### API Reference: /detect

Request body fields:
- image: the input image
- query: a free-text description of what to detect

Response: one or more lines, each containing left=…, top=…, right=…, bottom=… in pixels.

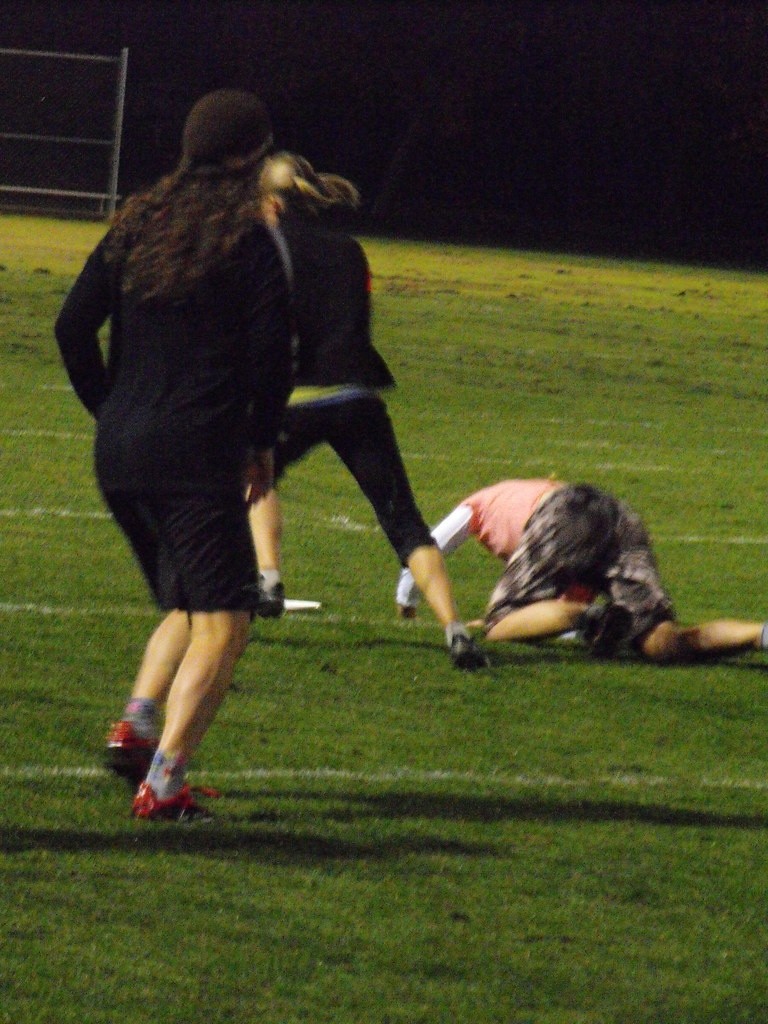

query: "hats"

left=177, top=87, right=275, bottom=180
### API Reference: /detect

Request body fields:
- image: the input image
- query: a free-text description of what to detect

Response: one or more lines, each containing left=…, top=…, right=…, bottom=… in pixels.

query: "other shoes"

left=582, top=605, right=631, bottom=662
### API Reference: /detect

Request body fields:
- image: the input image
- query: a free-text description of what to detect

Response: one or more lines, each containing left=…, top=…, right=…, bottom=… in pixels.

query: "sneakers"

left=105, top=723, right=221, bottom=801
left=129, top=783, right=214, bottom=822
left=257, top=574, right=285, bottom=620
left=450, top=633, right=491, bottom=670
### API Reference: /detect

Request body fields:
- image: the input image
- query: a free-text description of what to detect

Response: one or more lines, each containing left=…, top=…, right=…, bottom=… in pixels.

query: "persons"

left=398, top=477, right=767, bottom=666
left=246, top=150, right=484, bottom=672
left=57, top=89, right=292, bottom=819
